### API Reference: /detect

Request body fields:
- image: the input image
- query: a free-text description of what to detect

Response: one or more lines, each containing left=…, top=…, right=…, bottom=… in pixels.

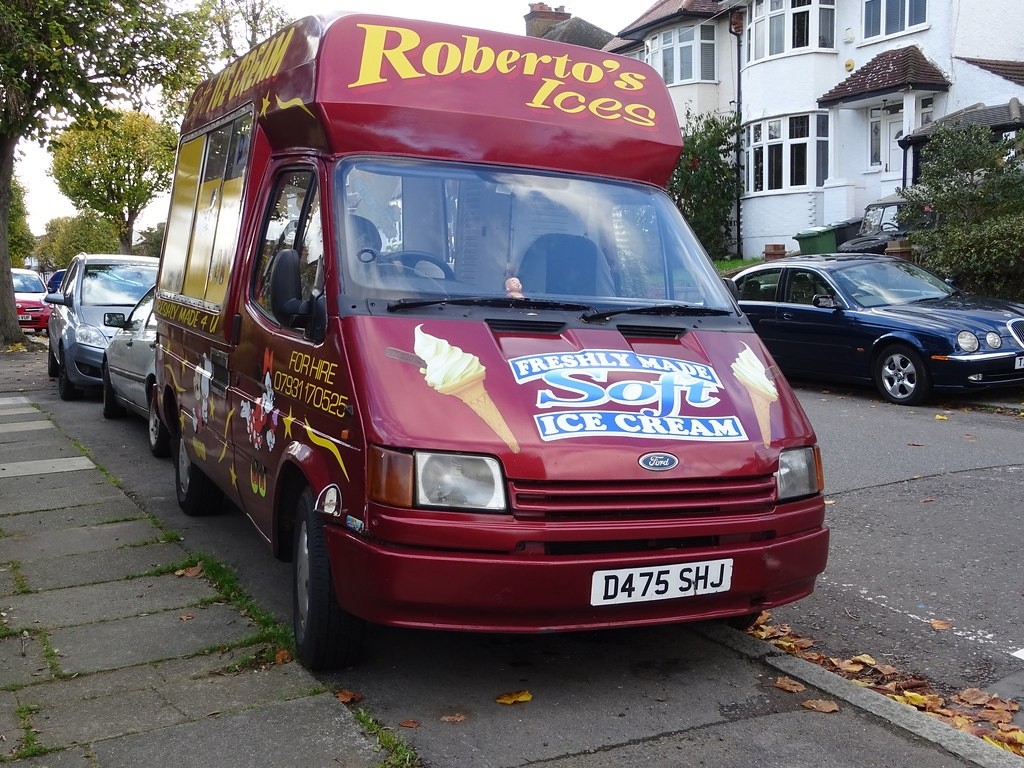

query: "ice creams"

left=385, top=324, right=520, bottom=454
left=730, top=341, right=779, bottom=450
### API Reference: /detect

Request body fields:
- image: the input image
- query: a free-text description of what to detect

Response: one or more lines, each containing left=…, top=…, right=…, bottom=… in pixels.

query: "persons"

left=505, top=278, right=523, bottom=299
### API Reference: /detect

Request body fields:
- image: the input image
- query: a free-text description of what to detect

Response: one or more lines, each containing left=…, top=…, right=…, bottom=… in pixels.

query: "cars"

left=45, top=269, right=67, bottom=294
left=102, top=282, right=177, bottom=457
left=729, top=252, right=1024, bottom=406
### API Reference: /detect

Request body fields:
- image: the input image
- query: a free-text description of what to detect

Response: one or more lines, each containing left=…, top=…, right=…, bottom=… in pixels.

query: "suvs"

left=48, top=251, right=161, bottom=402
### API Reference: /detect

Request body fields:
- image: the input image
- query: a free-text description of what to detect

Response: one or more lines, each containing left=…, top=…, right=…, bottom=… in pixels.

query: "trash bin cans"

left=792, top=224, right=840, bottom=254
left=828, top=216, right=870, bottom=245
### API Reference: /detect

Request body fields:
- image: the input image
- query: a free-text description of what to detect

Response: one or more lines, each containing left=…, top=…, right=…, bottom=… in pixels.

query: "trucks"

left=151, top=12, right=829, bottom=684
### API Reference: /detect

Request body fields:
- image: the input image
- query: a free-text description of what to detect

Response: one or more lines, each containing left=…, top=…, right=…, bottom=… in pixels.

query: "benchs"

left=741, top=280, right=778, bottom=301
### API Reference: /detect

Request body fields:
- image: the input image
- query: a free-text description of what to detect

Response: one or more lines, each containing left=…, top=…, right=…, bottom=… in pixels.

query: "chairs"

left=792, top=274, right=828, bottom=304
left=349, top=215, right=385, bottom=256
left=517, top=235, right=616, bottom=293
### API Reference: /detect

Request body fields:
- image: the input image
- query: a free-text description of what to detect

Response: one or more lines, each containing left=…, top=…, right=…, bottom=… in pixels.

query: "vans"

left=10, top=268, right=51, bottom=332
